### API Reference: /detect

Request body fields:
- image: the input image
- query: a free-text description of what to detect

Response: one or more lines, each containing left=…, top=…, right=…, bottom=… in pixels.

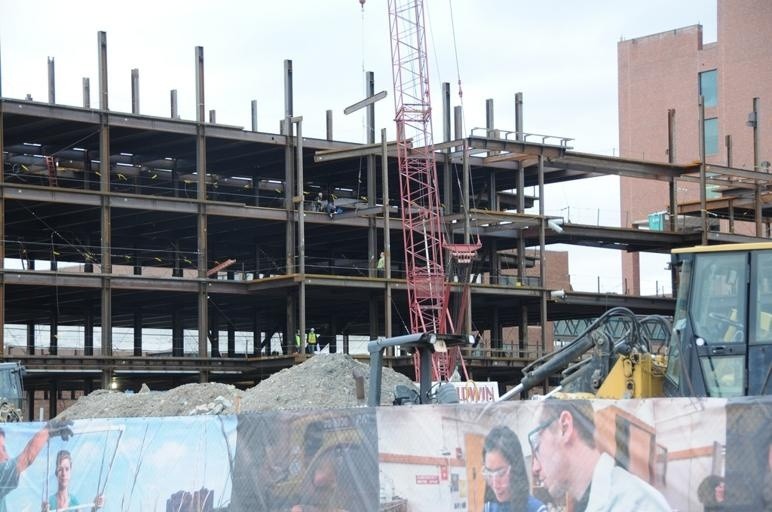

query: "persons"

left=313, top=192, right=323, bottom=212
left=481, top=426, right=547, bottom=512
left=307, top=327, right=318, bottom=353
left=0, top=420, right=73, bottom=512
left=295, top=329, right=300, bottom=353
left=377, top=251, right=385, bottom=278
left=528, top=400, right=670, bottom=512
left=41, top=450, right=104, bottom=512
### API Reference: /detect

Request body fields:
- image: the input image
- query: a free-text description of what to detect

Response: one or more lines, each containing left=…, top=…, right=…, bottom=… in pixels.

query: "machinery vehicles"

left=271, top=329, right=475, bottom=510
left=479, top=242, right=771, bottom=397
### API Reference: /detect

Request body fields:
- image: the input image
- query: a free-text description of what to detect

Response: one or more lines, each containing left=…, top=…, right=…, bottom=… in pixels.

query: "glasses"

left=528, top=416, right=560, bottom=455
left=478, top=467, right=505, bottom=482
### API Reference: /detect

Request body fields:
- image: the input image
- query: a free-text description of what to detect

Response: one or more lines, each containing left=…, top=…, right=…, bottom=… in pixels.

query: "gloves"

left=45, top=421, right=76, bottom=441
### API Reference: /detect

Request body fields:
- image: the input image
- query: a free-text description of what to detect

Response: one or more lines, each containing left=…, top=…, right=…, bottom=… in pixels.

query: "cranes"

left=360, top=0, right=499, bottom=402
